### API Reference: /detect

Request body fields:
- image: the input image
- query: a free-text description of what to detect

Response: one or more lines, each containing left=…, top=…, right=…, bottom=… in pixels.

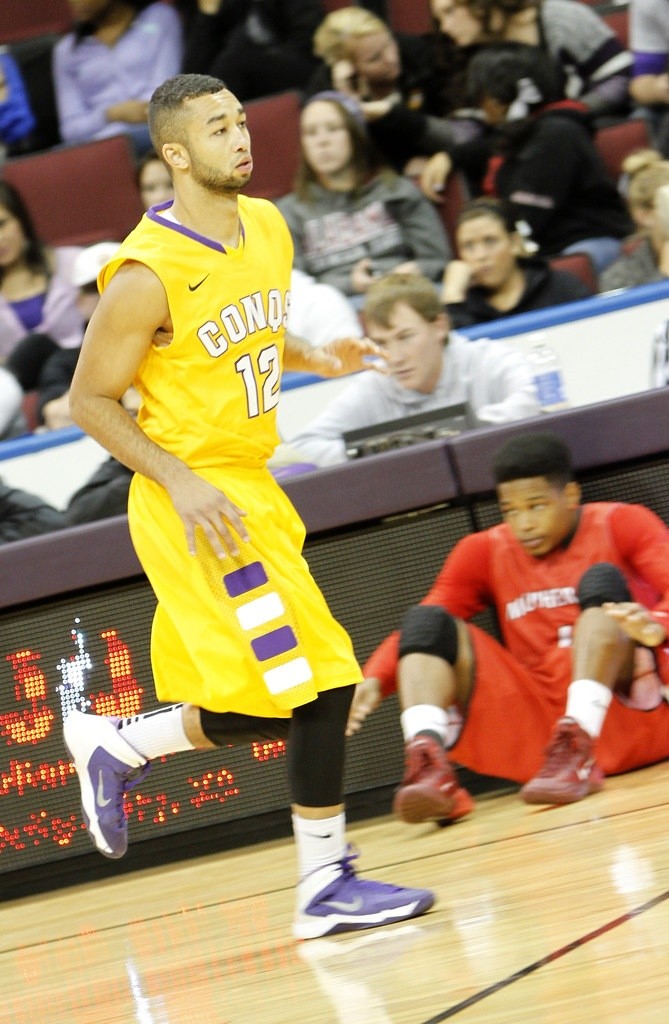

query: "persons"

left=1, top=1, right=669, bottom=541
left=67, top=73, right=435, bottom=943
left=344, top=433, right=668, bottom=826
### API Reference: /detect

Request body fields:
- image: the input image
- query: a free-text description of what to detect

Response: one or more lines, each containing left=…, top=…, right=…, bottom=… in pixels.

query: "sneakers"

left=522, top=716, right=603, bottom=803
left=62, top=711, right=151, bottom=859
left=397, top=737, right=475, bottom=828
left=293, top=843, right=435, bottom=940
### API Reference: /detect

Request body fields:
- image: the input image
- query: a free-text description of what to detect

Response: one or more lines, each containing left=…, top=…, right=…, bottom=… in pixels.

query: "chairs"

left=1, top=0, right=652, bottom=296
